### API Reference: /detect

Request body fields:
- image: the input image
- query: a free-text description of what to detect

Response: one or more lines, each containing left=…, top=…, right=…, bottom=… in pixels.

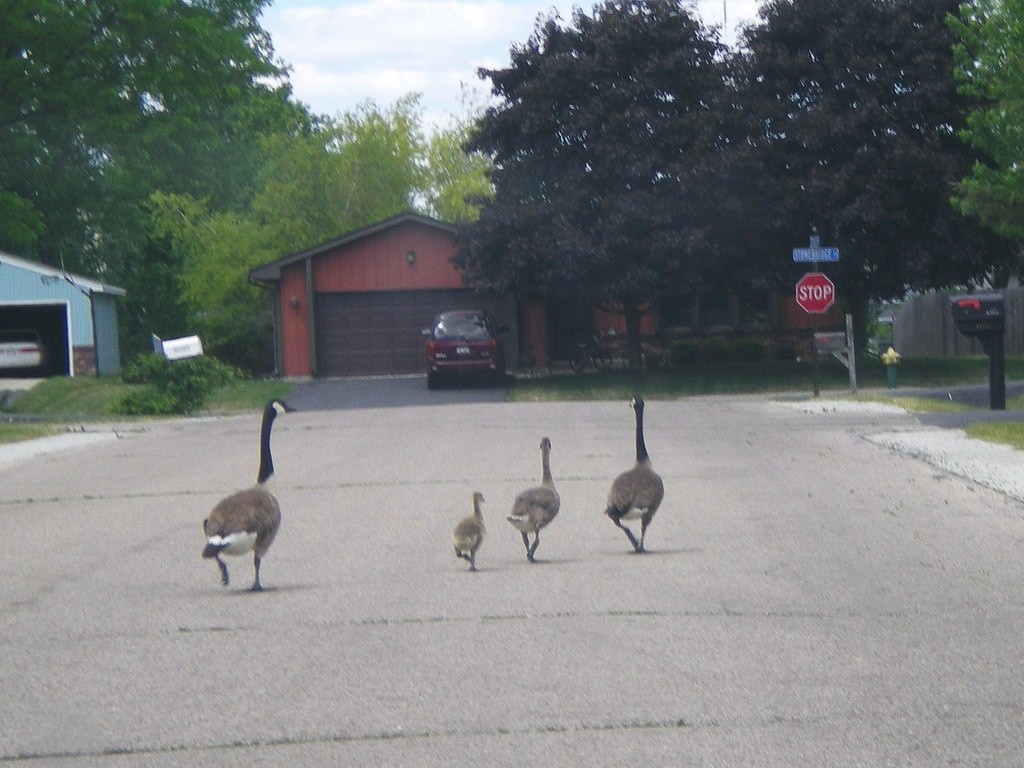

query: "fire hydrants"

left=881, top=346, right=903, bottom=390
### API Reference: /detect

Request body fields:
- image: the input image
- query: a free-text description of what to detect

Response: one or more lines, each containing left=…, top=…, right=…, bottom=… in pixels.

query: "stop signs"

left=795, top=273, right=835, bottom=314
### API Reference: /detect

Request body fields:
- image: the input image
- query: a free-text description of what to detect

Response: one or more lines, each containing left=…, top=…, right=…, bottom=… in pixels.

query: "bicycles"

left=567, top=340, right=612, bottom=374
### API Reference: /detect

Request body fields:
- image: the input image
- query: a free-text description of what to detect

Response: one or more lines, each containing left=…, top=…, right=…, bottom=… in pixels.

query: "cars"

left=0, top=330, right=54, bottom=376
left=421, top=309, right=510, bottom=392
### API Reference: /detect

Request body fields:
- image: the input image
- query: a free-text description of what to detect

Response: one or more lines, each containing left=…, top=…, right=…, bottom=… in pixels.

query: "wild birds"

left=201, top=398, right=296, bottom=593
left=454, top=491, right=487, bottom=573
left=604, top=394, right=665, bottom=554
left=507, top=436, right=561, bottom=564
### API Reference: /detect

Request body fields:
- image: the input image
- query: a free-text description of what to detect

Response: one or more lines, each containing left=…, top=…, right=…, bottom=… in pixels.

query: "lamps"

left=407, top=251, right=414, bottom=265
left=290, top=294, right=298, bottom=309
